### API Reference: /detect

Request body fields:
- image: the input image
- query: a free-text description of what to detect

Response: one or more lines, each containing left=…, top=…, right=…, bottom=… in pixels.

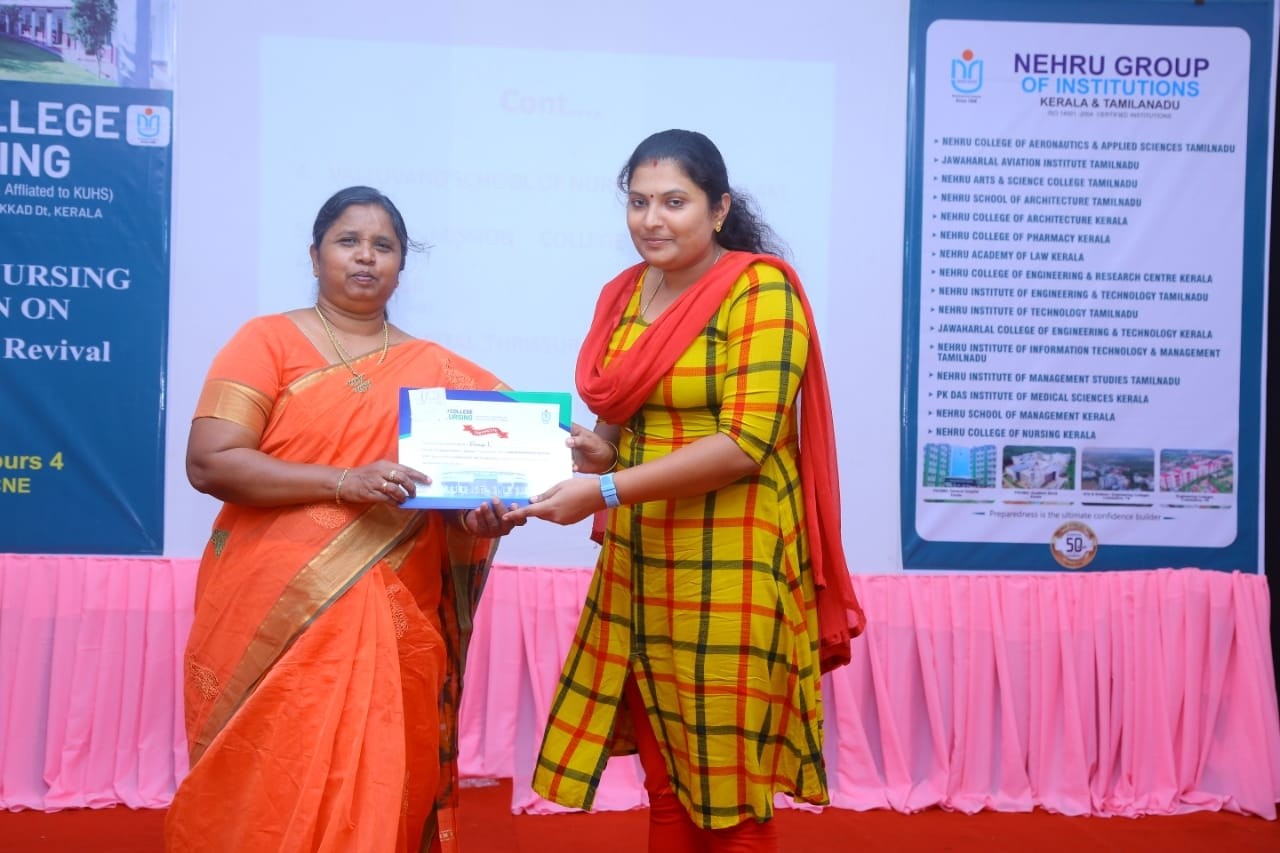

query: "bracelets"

left=335, top=468, right=348, bottom=505
left=600, top=472, right=620, bottom=509
left=459, top=509, right=473, bottom=536
left=600, top=440, right=619, bottom=474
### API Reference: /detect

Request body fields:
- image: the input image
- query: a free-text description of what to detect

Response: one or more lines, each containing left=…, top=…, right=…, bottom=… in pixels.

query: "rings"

left=389, top=470, right=396, bottom=481
left=383, top=481, right=388, bottom=492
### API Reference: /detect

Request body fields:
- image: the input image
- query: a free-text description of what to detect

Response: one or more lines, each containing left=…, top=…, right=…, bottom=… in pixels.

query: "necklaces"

left=314, top=303, right=389, bottom=392
left=329, top=327, right=354, bottom=359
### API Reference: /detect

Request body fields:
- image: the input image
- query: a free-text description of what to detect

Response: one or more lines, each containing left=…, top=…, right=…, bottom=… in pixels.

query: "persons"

left=185, top=186, right=526, bottom=853
left=503, top=131, right=865, bottom=852
